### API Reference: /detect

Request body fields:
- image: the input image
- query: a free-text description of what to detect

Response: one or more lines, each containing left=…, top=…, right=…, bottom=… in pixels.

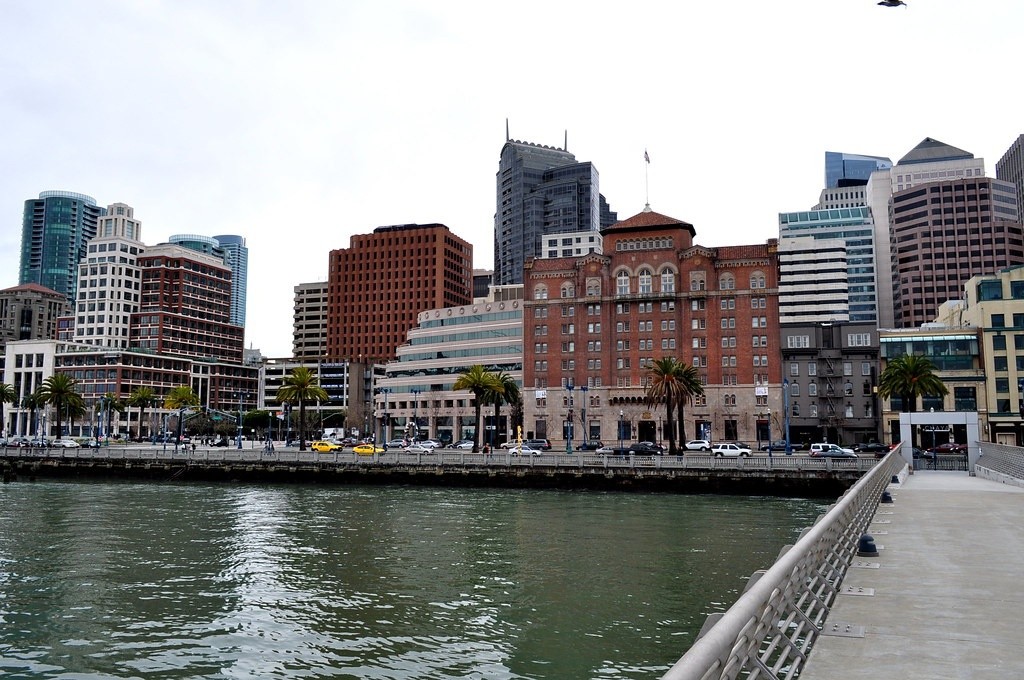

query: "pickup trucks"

left=761, top=440, right=805, bottom=452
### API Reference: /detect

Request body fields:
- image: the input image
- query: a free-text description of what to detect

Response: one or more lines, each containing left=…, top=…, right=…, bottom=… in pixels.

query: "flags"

left=644, top=149, right=651, bottom=164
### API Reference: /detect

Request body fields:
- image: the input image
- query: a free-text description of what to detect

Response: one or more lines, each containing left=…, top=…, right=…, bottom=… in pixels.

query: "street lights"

left=42, top=413, right=46, bottom=445
left=930, top=407, right=937, bottom=470
left=489, top=411, right=493, bottom=458
left=321, top=402, right=343, bottom=434
left=620, top=409, right=624, bottom=461
left=101, top=393, right=118, bottom=447
left=766, top=408, right=773, bottom=468
left=96, top=412, right=101, bottom=450
left=233, top=391, right=251, bottom=449
left=148, top=399, right=164, bottom=446
left=269, top=412, right=272, bottom=450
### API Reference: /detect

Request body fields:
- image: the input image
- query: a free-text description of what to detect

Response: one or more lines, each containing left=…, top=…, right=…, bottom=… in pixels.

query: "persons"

left=17, top=442, right=53, bottom=453
left=180, top=435, right=214, bottom=455
left=483, top=443, right=490, bottom=463
left=98, top=434, right=122, bottom=446
left=676, top=446, right=684, bottom=465
left=263, top=442, right=275, bottom=455
left=598, top=442, right=604, bottom=448
left=383, top=440, right=388, bottom=451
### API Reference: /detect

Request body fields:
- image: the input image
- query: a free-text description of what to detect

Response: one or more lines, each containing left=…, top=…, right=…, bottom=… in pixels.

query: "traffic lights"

left=581, top=386, right=588, bottom=443
left=566, top=385, right=574, bottom=454
left=381, top=417, right=386, bottom=426
left=410, top=389, right=421, bottom=439
left=379, top=387, right=392, bottom=445
left=215, top=412, right=218, bottom=421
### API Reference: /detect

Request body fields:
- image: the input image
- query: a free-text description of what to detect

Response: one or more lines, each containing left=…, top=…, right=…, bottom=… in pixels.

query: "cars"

left=0, top=437, right=100, bottom=448
left=290, top=439, right=552, bottom=457
left=809, top=441, right=967, bottom=464
left=210, top=438, right=228, bottom=447
left=130, top=436, right=190, bottom=444
left=576, top=441, right=753, bottom=460
